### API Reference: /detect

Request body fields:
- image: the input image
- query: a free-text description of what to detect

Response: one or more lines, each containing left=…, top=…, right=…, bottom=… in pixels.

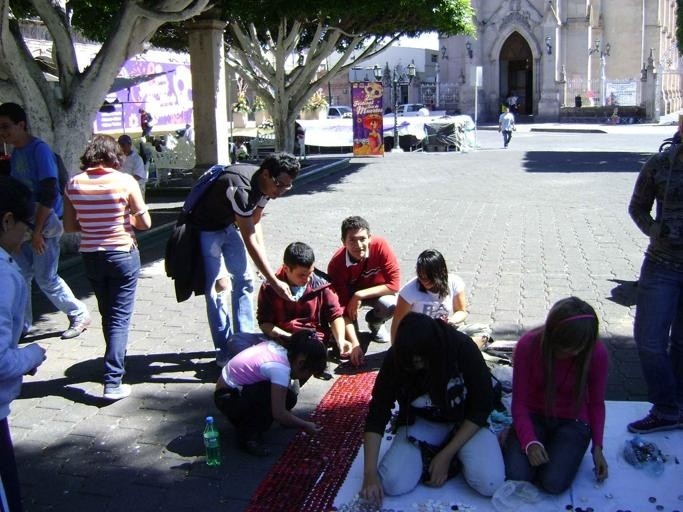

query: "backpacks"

left=54, top=152, right=70, bottom=195
left=179, top=165, right=226, bottom=217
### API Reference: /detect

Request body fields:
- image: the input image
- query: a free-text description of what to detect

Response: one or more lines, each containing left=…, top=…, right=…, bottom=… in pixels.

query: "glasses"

left=272, top=176, right=292, bottom=191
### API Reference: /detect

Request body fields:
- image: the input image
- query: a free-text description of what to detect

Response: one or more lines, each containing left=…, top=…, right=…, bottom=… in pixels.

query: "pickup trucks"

left=382, top=103, right=448, bottom=118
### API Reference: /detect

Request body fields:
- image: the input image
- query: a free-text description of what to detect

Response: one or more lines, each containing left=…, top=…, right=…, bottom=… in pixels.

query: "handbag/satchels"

left=493, top=381, right=512, bottom=412
left=408, top=426, right=463, bottom=484
left=147, top=115, right=157, bottom=127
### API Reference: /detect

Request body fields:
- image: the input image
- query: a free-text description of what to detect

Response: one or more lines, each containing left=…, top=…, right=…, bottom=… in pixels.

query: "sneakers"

left=62, top=315, right=91, bottom=338
left=368, top=322, right=391, bottom=343
left=244, top=441, right=272, bottom=457
left=315, top=368, right=333, bottom=379
left=216, top=348, right=231, bottom=367
left=628, top=412, right=679, bottom=434
left=104, top=383, right=131, bottom=400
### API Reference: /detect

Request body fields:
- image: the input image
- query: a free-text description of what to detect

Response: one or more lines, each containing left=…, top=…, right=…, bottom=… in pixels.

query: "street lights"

left=374, top=62, right=419, bottom=154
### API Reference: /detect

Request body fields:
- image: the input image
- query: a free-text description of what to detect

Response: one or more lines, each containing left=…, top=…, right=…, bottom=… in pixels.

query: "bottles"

left=203, top=416, right=223, bottom=467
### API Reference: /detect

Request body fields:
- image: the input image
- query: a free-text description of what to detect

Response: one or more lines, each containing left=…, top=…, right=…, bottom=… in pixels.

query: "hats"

left=118, top=135, right=132, bottom=144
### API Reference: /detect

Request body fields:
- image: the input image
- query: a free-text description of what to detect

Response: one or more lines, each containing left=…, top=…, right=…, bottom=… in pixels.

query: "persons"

left=182, top=122, right=194, bottom=145
left=63, top=135, right=151, bottom=400
left=0, top=174, right=46, bottom=511
left=325, top=214, right=402, bottom=369
left=507, top=90, right=520, bottom=122
left=212, top=329, right=325, bottom=451
left=626, top=104, right=682, bottom=434
left=137, top=107, right=153, bottom=138
left=389, top=248, right=491, bottom=341
left=233, top=139, right=247, bottom=156
left=255, top=240, right=353, bottom=372
left=0, top=101, right=92, bottom=339
left=164, top=150, right=302, bottom=369
left=498, top=107, right=516, bottom=148
left=357, top=312, right=504, bottom=507
left=499, top=295, right=608, bottom=495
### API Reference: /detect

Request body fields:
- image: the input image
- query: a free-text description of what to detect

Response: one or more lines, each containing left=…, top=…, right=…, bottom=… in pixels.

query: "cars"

left=327, top=105, right=352, bottom=119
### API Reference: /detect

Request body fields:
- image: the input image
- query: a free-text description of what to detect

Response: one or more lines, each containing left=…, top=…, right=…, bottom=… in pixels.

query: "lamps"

left=545, top=37, right=552, bottom=54
left=441, top=45, right=448, bottom=60
left=458, top=71, right=465, bottom=84
left=589, top=39, right=600, bottom=55
left=466, top=40, right=472, bottom=58
left=604, top=42, right=610, bottom=57
